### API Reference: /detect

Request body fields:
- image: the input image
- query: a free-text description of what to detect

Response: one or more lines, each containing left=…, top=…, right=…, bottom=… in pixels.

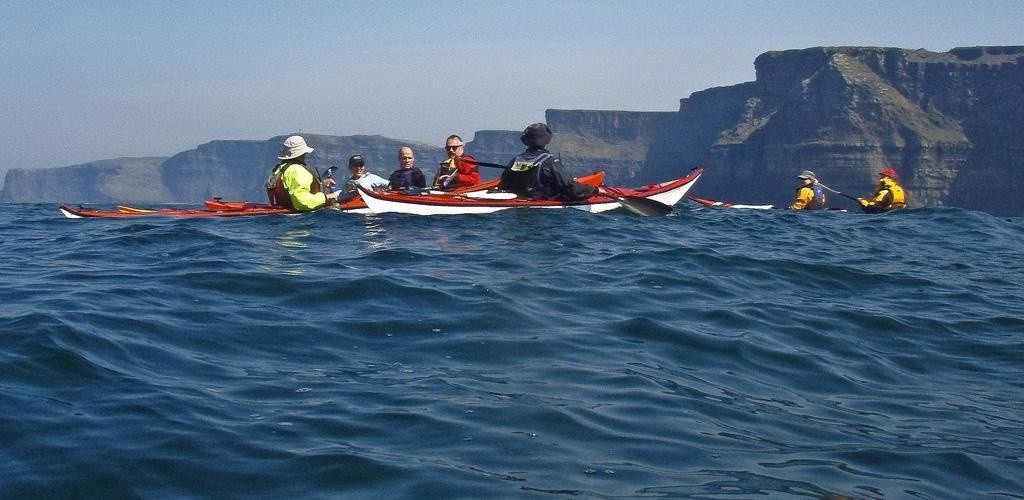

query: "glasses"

left=445, top=143, right=463, bottom=152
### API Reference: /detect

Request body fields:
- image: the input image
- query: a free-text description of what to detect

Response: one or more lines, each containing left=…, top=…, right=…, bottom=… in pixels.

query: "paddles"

left=322, top=165, right=340, bottom=210
left=438, top=169, right=458, bottom=188
left=461, top=159, right=673, bottom=216
left=818, top=183, right=859, bottom=203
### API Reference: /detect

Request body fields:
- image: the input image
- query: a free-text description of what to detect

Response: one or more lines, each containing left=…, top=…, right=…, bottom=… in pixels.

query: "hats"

left=348, top=154, right=364, bottom=164
left=276, top=135, right=314, bottom=160
left=797, top=170, right=816, bottom=180
left=519, top=122, right=553, bottom=148
left=879, top=167, right=898, bottom=181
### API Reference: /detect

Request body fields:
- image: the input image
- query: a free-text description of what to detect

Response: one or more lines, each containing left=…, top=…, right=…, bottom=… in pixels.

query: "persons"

left=336, top=155, right=391, bottom=202
left=265, top=135, right=336, bottom=211
left=431, top=135, right=464, bottom=190
left=786, top=170, right=825, bottom=210
left=387, top=147, right=425, bottom=188
left=857, top=169, right=908, bottom=214
left=499, top=123, right=599, bottom=200
left=438, top=155, right=480, bottom=185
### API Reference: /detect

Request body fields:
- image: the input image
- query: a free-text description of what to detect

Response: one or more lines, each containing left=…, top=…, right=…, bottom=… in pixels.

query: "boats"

left=688, top=193, right=845, bottom=213
